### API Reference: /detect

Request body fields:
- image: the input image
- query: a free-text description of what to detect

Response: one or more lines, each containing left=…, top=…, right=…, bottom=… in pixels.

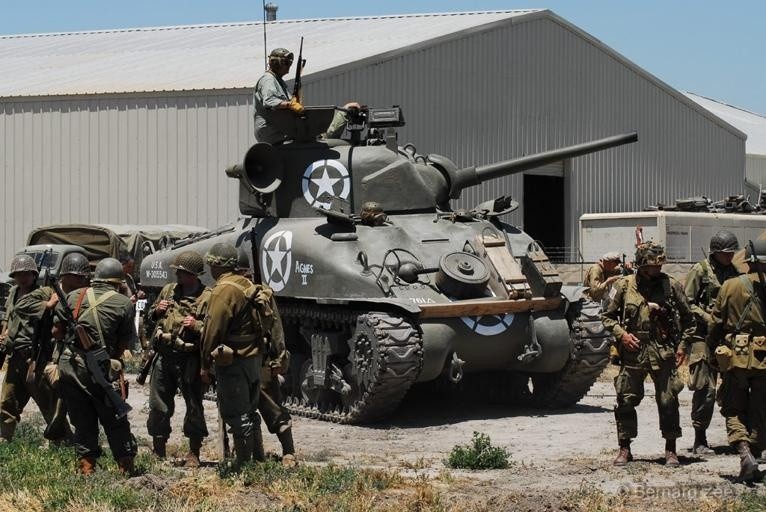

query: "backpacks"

left=217, top=276, right=274, bottom=354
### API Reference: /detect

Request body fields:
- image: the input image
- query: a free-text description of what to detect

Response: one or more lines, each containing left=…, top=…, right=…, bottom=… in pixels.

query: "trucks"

left=0, top=222, right=210, bottom=326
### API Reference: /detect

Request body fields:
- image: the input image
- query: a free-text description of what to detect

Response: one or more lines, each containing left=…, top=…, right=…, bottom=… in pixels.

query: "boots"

left=614, top=437, right=633, bottom=466
left=277, top=426, right=296, bottom=471
left=732, top=440, right=758, bottom=483
left=692, top=429, right=715, bottom=454
left=183, top=437, right=203, bottom=467
left=665, top=439, right=680, bottom=468
left=153, top=434, right=166, bottom=460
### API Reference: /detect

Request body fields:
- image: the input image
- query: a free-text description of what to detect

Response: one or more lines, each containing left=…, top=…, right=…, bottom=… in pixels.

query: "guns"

left=136, top=347, right=156, bottom=384
left=217, top=416, right=231, bottom=461
left=48, top=273, right=133, bottom=420
left=249, top=228, right=281, bottom=395
left=749, top=240, right=766, bottom=299
left=293, top=36, right=306, bottom=101
left=1, top=252, right=47, bottom=368
left=618, top=254, right=626, bottom=326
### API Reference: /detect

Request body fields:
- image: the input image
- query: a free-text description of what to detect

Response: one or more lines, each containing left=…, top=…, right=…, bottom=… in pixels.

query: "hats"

left=602, top=252, right=621, bottom=264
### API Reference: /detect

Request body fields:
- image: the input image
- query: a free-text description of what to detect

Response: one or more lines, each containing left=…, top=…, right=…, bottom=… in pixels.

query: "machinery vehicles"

left=576, top=188, right=766, bottom=263
left=127, top=0, right=640, bottom=428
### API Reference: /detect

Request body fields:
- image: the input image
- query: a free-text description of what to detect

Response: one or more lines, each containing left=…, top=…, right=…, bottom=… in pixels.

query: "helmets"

left=169, top=250, right=205, bottom=276
left=741, top=242, right=752, bottom=263
left=92, top=258, right=123, bottom=284
left=751, top=233, right=766, bottom=264
left=8, top=254, right=39, bottom=281
left=268, top=48, right=293, bottom=69
left=59, top=252, right=90, bottom=276
left=634, top=241, right=666, bottom=267
left=708, top=229, right=739, bottom=255
left=234, top=247, right=250, bottom=270
left=205, top=242, right=237, bottom=268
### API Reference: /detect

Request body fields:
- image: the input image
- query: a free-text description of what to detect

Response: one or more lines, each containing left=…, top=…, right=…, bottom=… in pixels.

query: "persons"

left=0, top=242, right=295, bottom=478
left=583, top=230, right=766, bottom=479
left=321, top=102, right=361, bottom=140
left=253, top=48, right=304, bottom=146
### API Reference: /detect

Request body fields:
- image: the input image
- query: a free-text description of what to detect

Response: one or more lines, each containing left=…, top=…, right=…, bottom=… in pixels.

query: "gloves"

left=289, top=97, right=304, bottom=114
